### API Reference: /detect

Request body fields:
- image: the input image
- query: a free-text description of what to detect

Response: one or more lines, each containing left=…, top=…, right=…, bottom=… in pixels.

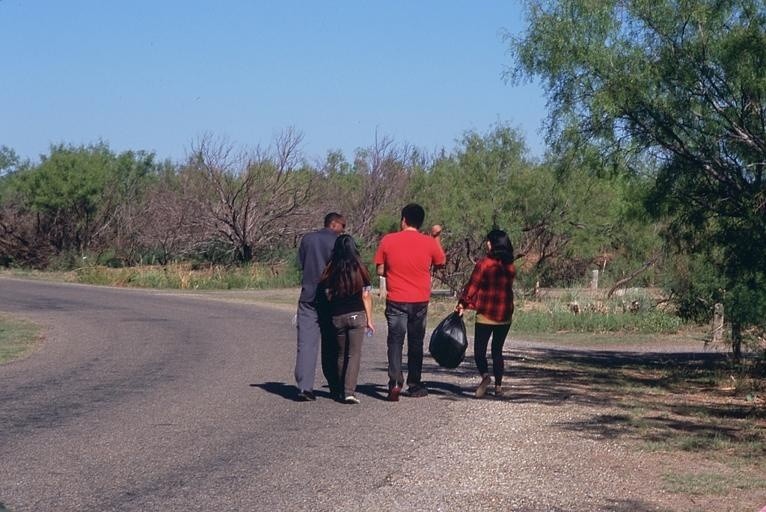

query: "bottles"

left=366, top=327, right=373, bottom=339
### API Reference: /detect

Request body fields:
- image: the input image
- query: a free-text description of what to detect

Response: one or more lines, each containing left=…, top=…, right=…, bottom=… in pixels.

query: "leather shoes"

left=297, top=390, right=316, bottom=401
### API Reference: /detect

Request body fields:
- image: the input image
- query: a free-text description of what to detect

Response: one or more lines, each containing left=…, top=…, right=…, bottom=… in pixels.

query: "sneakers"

left=345, top=395, right=360, bottom=404
left=388, top=379, right=402, bottom=401
left=476, top=376, right=491, bottom=398
left=495, top=390, right=504, bottom=397
left=409, top=387, right=428, bottom=397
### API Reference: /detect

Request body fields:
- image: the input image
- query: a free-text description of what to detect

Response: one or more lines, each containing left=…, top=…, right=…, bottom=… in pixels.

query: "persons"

left=455, top=230, right=515, bottom=398
left=294, top=212, right=345, bottom=401
left=319, top=235, right=375, bottom=403
left=374, top=203, right=447, bottom=401
